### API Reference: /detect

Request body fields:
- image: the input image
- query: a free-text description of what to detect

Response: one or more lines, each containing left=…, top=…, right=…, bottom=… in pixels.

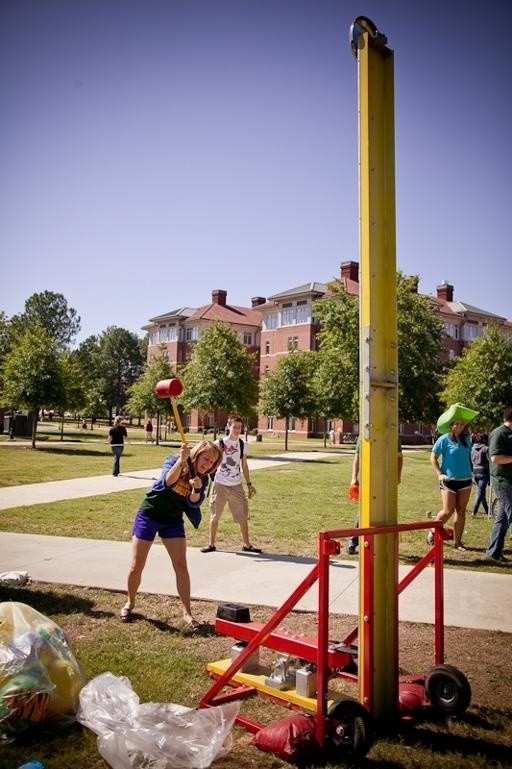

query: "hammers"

left=155, top=379, right=195, bottom=483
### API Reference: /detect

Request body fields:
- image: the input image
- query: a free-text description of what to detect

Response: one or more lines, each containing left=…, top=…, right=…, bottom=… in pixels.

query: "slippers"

left=189, top=620, right=200, bottom=632
left=120, top=608, right=131, bottom=622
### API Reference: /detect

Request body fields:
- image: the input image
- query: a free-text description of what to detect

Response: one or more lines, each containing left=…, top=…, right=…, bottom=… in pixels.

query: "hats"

left=436, top=402, right=478, bottom=434
left=492, top=554, right=507, bottom=562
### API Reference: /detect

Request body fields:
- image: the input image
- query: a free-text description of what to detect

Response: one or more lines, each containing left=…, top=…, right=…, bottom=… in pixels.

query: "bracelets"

left=247, top=483, right=251, bottom=486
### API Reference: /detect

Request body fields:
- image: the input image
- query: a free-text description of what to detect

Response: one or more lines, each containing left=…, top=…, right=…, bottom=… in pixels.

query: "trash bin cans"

left=328, top=430, right=340, bottom=445
left=4, top=415, right=14, bottom=433
left=257, top=434, right=262, bottom=441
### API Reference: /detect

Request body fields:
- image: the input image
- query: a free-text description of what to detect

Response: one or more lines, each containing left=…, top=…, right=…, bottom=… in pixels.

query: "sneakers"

left=454, top=545, right=466, bottom=551
left=242, top=545, right=261, bottom=554
left=347, top=546, right=354, bottom=554
left=427, top=531, right=434, bottom=544
left=201, top=546, right=216, bottom=552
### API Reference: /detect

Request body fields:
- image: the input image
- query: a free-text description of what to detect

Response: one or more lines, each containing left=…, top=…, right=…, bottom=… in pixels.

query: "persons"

left=347, top=415, right=511, bottom=562
left=82, top=419, right=88, bottom=428
left=109, top=417, right=131, bottom=477
left=145, top=420, right=155, bottom=443
left=330, top=429, right=335, bottom=445
left=201, top=416, right=262, bottom=553
left=120, top=440, right=223, bottom=632
